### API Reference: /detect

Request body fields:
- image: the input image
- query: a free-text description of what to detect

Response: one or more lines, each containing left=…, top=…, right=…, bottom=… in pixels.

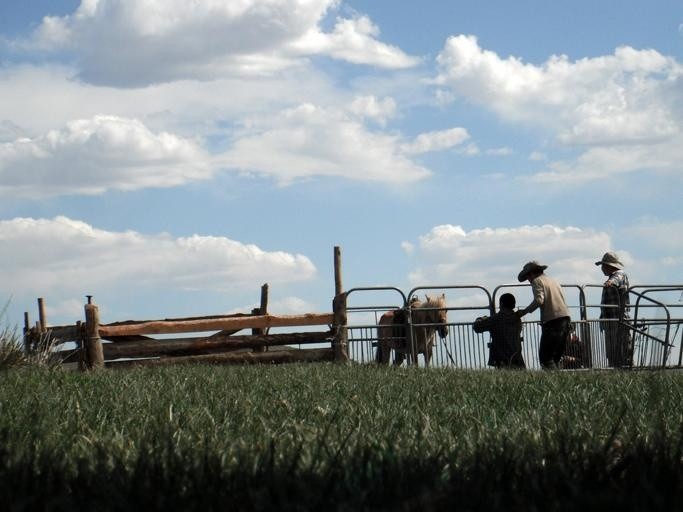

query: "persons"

left=558, top=322, right=585, bottom=369
left=516, top=259, right=571, bottom=369
left=594, top=250, right=635, bottom=368
left=473, top=292, right=527, bottom=368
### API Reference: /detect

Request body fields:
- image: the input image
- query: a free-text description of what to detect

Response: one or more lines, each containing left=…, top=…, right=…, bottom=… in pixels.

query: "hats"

left=594, top=251, right=625, bottom=270
left=517, top=260, right=548, bottom=282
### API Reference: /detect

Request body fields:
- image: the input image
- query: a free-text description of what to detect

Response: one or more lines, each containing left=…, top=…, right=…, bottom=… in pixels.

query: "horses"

left=373, top=292, right=449, bottom=368
